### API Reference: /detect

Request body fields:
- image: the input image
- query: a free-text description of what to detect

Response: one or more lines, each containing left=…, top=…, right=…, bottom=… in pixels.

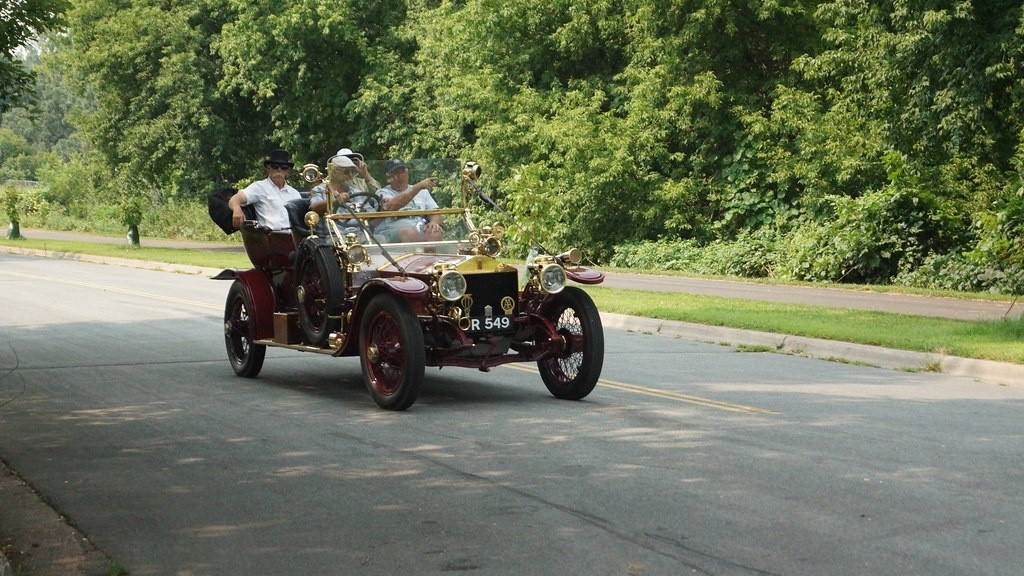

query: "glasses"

left=336, top=168, right=356, bottom=175
left=269, top=163, right=289, bottom=170
left=392, top=168, right=409, bottom=176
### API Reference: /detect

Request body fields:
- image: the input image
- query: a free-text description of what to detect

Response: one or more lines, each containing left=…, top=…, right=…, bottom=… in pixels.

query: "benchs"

left=238, top=190, right=311, bottom=220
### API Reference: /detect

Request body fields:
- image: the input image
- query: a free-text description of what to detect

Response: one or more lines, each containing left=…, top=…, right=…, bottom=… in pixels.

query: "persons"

left=229, top=150, right=303, bottom=308
left=373, top=155, right=446, bottom=243
left=310, top=148, right=389, bottom=244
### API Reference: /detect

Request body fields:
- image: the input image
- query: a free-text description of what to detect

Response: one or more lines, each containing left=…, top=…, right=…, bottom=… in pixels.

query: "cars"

left=208, top=159, right=606, bottom=412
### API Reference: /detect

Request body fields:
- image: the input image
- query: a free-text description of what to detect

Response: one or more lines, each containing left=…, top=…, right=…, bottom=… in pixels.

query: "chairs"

left=283, top=195, right=334, bottom=252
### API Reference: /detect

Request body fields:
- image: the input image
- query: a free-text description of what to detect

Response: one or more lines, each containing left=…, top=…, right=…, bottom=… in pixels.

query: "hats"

left=383, top=158, right=410, bottom=174
left=264, top=149, right=294, bottom=170
left=331, top=156, right=357, bottom=168
left=337, top=149, right=352, bottom=155
